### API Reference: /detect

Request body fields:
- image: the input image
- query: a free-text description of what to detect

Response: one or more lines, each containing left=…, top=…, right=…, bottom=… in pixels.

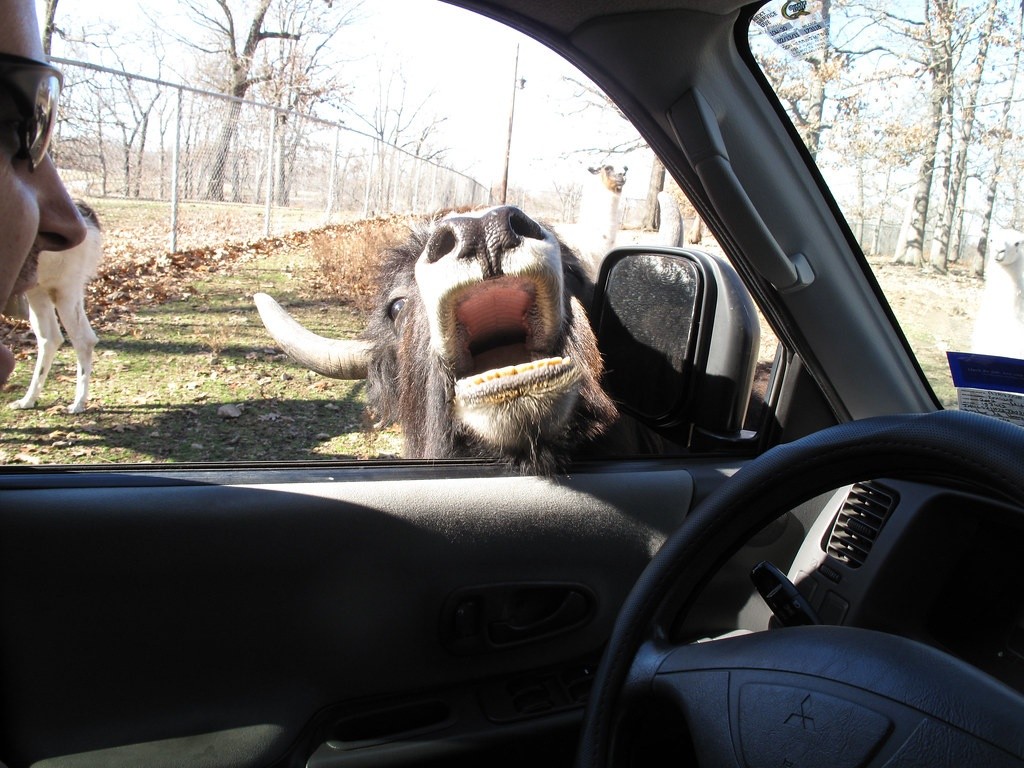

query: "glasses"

left=0, top=52, right=63, bottom=173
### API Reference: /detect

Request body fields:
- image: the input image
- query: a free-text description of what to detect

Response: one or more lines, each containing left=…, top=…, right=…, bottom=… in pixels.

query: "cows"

left=253, top=191, right=683, bottom=478
left=577, top=163, right=629, bottom=251
left=4, top=195, right=106, bottom=414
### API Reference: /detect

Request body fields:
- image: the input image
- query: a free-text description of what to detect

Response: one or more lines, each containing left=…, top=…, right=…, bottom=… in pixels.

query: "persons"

left=0, top=0, right=88, bottom=385
